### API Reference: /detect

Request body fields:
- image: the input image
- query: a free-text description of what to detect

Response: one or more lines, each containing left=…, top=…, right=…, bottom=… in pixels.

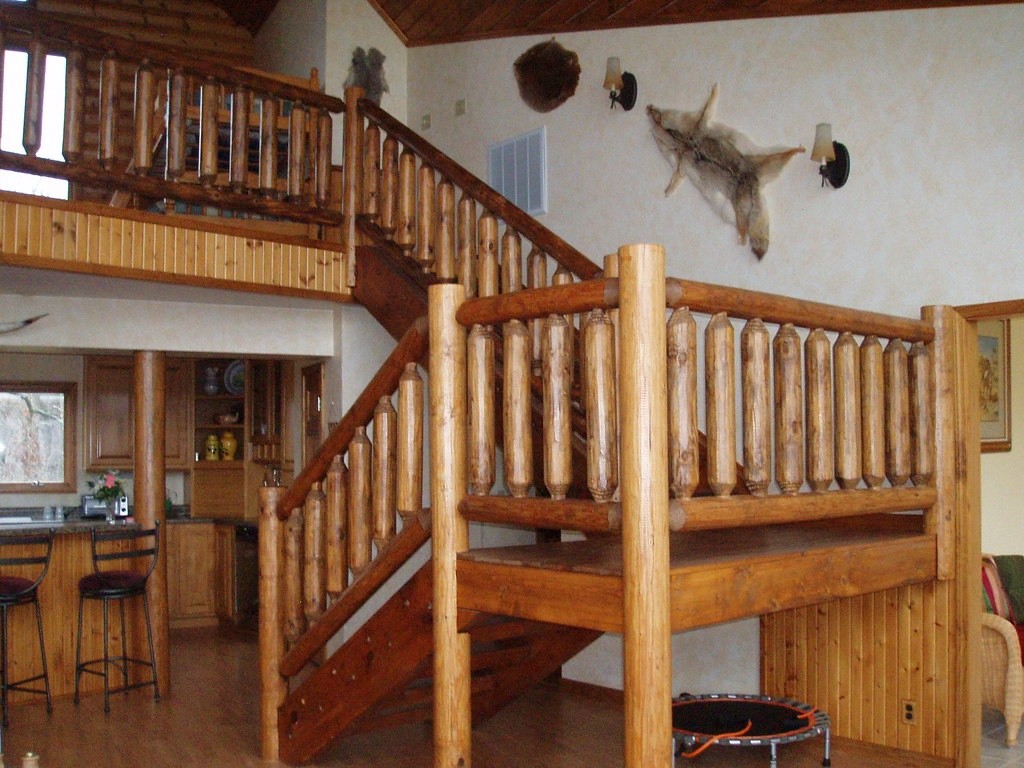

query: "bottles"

left=203, top=436, right=220, bottom=460
left=218, top=430, right=238, bottom=461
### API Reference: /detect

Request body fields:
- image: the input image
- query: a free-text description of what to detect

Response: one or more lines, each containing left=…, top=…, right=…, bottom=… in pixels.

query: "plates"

left=222, top=359, right=248, bottom=395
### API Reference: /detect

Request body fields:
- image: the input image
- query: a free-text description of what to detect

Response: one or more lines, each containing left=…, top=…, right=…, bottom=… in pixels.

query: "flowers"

left=88, top=472, right=128, bottom=506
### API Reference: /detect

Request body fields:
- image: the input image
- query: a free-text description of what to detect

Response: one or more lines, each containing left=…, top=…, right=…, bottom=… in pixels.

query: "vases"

left=105, top=505, right=116, bottom=525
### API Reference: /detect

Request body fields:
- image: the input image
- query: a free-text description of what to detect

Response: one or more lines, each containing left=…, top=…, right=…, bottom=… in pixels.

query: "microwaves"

left=82, top=494, right=128, bottom=516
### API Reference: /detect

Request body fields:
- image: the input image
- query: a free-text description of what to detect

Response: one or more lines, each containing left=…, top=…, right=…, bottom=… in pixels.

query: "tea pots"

left=213, top=413, right=240, bottom=425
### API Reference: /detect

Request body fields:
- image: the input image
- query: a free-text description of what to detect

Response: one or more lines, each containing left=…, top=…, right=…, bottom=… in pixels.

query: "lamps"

left=603, top=56, right=637, bottom=111
left=809, top=123, right=851, bottom=188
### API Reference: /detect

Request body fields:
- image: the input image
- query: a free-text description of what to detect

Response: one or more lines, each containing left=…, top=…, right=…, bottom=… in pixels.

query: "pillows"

left=994, top=555, right=1024, bottom=625
left=982, top=556, right=1016, bottom=625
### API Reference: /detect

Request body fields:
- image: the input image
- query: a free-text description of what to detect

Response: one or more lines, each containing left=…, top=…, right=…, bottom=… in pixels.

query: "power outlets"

left=902, top=700, right=918, bottom=725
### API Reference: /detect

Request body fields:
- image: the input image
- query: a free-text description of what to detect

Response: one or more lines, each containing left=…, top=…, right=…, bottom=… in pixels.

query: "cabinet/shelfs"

left=81, top=353, right=294, bottom=630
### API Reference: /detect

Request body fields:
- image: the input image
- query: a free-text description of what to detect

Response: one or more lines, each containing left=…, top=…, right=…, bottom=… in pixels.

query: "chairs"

left=72, top=522, right=163, bottom=714
left=981, top=553, right=1024, bottom=747
left=0, top=527, right=56, bottom=730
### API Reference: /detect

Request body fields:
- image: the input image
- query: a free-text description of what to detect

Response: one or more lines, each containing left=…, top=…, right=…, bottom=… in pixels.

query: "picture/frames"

left=973, top=318, right=1013, bottom=453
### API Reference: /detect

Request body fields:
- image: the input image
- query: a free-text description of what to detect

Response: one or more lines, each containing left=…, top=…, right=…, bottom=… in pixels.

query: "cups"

left=53, top=505, right=65, bottom=522
left=43, top=506, right=55, bottom=521
